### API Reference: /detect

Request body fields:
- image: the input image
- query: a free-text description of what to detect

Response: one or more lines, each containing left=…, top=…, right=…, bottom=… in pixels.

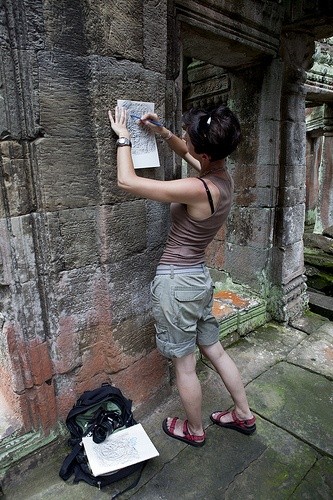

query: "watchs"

left=115, top=137, right=133, bottom=150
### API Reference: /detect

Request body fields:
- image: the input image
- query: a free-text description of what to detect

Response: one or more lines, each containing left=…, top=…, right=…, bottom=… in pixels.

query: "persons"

left=108, top=103, right=256, bottom=449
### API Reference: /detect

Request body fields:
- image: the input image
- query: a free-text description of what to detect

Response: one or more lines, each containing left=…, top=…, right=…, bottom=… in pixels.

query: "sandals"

left=210, top=410, right=256, bottom=434
left=162, top=417, right=206, bottom=446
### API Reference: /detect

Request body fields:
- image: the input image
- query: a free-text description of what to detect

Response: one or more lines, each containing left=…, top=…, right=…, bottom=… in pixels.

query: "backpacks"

left=59, top=383, right=147, bottom=500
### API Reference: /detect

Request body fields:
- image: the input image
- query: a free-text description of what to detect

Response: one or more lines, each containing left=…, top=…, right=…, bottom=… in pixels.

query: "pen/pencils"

left=130, top=115, right=163, bottom=127
